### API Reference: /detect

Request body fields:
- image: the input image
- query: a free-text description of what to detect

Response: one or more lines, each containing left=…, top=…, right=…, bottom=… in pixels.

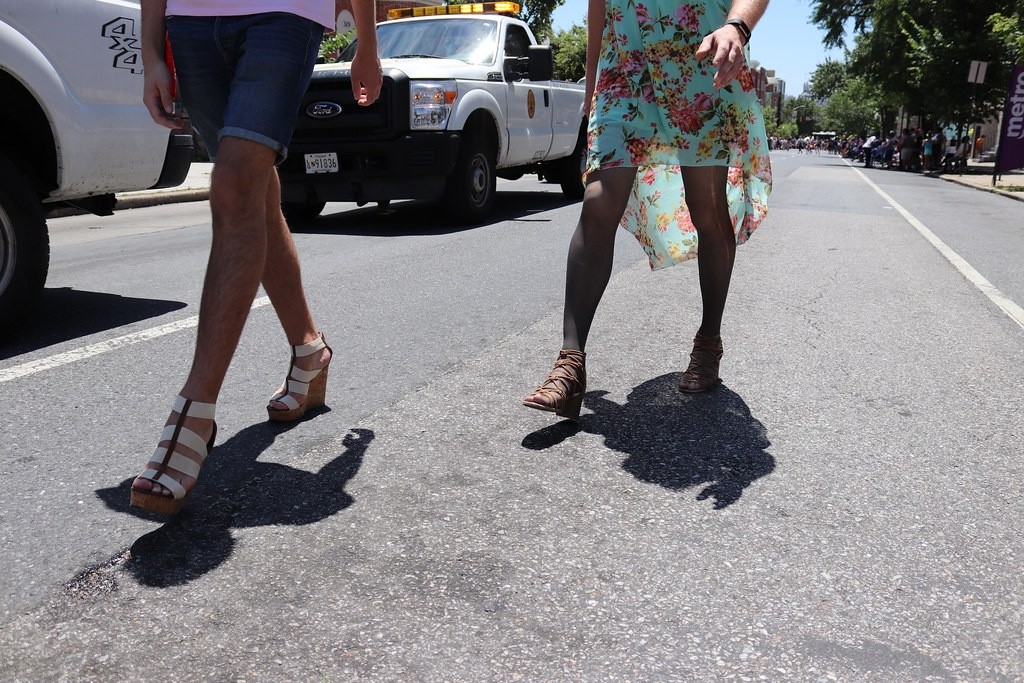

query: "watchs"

left=725, top=19, right=751, bottom=47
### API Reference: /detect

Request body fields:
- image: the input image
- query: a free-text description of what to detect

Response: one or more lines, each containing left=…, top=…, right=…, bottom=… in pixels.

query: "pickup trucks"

left=271, top=1, right=591, bottom=231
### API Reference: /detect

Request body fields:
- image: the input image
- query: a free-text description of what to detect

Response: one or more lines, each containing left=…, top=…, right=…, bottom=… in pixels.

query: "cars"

left=0, top=1, right=194, bottom=348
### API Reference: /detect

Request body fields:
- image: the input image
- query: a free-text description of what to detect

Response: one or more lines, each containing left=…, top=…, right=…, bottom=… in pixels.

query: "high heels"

left=127, top=394, right=217, bottom=518
left=680, top=331, right=724, bottom=392
left=268, top=336, right=333, bottom=420
left=524, top=349, right=588, bottom=417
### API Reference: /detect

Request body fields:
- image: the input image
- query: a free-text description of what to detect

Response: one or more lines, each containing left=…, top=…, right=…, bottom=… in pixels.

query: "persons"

left=764, top=126, right=986, bottom=174
left=128, top=0, right=387, bottom=516
left=523, top=0, right=771, bottom=416
